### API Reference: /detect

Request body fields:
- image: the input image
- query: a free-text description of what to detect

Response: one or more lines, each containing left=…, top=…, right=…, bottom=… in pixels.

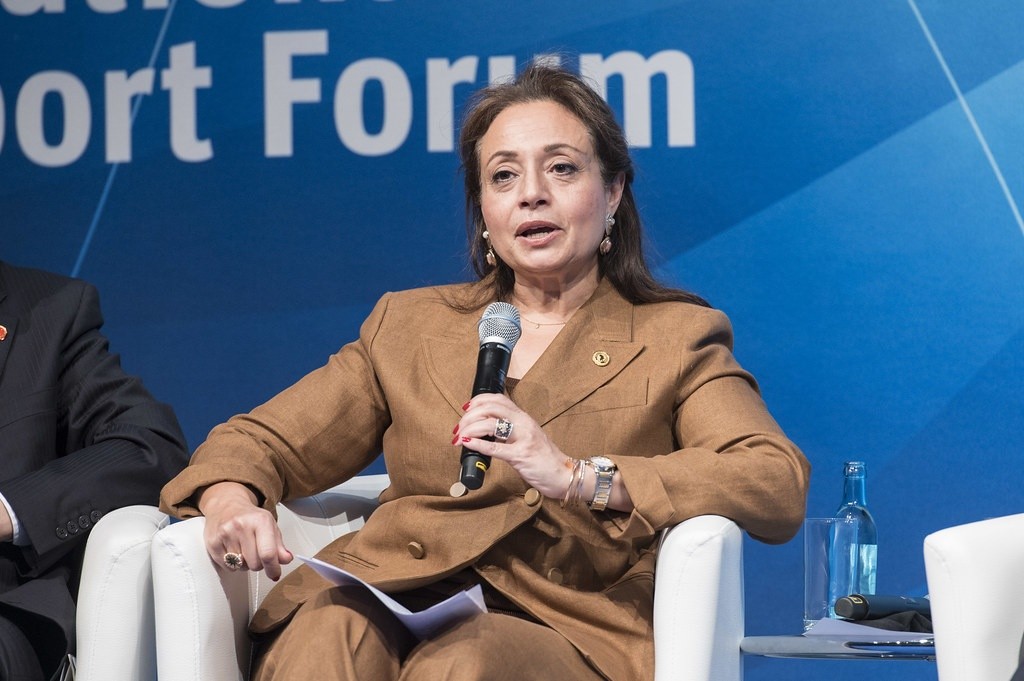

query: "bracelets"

left=560, top=459, right=587, bottom=508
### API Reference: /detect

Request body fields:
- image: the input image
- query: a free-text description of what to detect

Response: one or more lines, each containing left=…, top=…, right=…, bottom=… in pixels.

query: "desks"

left=742, top=635, right=936, bottom=663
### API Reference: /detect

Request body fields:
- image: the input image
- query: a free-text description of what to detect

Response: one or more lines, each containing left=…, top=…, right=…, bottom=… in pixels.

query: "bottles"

left=828, top=461, right=879, bottom=620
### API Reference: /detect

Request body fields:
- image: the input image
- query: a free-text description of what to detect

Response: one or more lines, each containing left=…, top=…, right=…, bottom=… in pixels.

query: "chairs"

left=151, top=474, right=745, bottom=681
left=75, top=506, right=170, bottom=681
left=923, top=512, right=1024, bottom=681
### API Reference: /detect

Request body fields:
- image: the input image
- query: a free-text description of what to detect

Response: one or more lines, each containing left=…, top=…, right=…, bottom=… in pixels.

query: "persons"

left=159, top=67, right=811, bottom=681
left=0, top=261, right=190, bottom=681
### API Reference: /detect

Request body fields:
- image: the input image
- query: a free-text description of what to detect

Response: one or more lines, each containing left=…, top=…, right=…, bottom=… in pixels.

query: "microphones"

left=835, top=593, right=932, bottom=621
left=460, top=302, right=522, bottom=490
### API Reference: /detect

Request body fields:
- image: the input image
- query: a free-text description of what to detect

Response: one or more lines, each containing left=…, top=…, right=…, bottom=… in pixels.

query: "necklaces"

left=521, top=316, right=566, bottom=328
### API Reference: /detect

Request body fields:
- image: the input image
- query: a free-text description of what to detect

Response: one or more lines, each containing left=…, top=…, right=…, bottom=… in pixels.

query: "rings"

left=224, top=553, right=243, bottom=569
left=494, top=418, right=513, bottom=439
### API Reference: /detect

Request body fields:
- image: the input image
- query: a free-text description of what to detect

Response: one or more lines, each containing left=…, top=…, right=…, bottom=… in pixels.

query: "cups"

left=803, top=517, right=858, bottom=632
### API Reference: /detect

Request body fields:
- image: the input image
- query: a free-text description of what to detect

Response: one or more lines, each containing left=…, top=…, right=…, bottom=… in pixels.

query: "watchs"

left=587, top=455, right=617, bottom=511
